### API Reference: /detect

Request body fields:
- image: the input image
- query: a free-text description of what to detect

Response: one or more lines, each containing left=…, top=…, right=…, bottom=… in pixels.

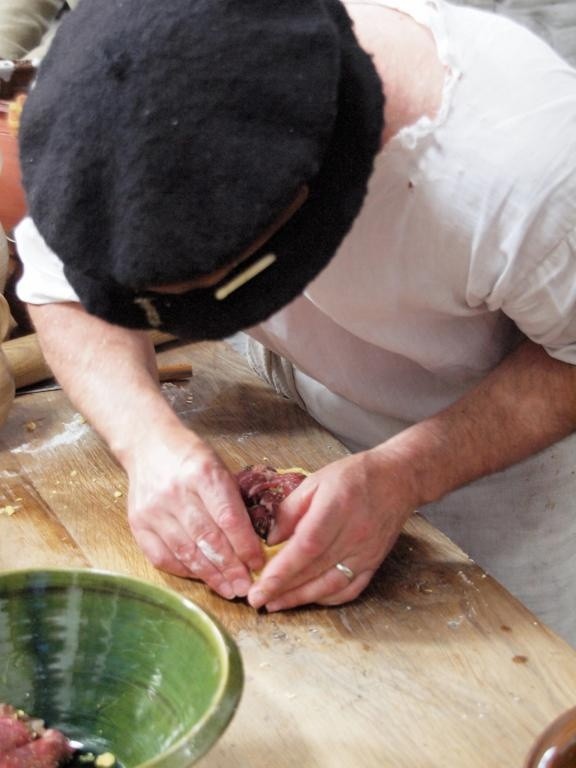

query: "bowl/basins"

left=0, top=567, right=243, bottom=768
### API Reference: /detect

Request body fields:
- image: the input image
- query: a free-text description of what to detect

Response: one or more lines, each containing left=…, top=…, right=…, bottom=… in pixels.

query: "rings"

left=336, top=562, right=355, bottom=581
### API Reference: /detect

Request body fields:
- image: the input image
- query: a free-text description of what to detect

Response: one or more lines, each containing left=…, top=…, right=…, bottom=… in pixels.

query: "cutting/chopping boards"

left=0, top=336, right=576, bottom=768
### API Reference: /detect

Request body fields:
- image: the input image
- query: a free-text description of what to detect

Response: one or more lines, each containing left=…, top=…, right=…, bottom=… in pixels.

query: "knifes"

left=14, top=363, right=192, bottom=395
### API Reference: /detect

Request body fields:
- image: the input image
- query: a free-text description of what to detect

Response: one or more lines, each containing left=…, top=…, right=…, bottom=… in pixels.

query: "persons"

left=13, top=0, right=576, bottom=610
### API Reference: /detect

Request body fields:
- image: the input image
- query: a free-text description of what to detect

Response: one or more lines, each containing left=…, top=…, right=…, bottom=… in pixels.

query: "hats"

left=14, top=1, right=388, bottom=339
